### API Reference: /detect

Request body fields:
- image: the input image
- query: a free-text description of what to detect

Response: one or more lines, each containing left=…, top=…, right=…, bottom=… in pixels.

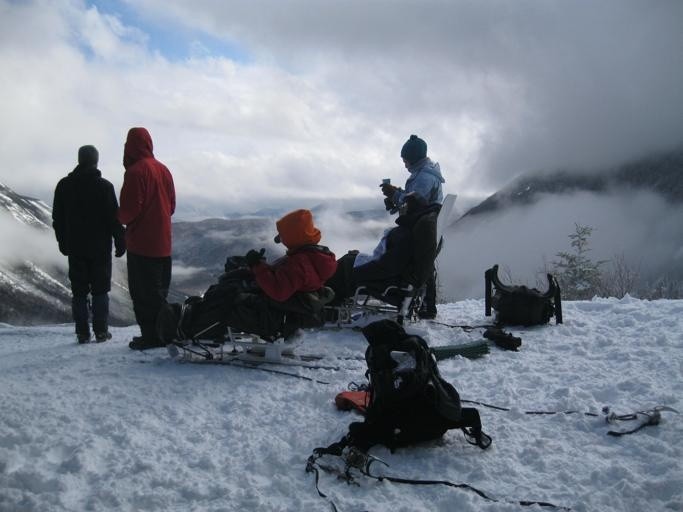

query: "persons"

left=163, top=210, right=338, bottom=345
left=117, top=127, right=176, bottom=350
left=381, top=135, right=445, bottom=319
left=322, top=190, right=431, bottom=322
left=52, top=145, right=127, bottom=344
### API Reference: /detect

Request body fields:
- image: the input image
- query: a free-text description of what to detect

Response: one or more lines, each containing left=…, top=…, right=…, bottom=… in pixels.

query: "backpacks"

left=344, top=319, right=481, bottom=449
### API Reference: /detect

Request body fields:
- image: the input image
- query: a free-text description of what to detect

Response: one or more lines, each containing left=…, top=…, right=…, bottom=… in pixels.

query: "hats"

left=401, top=134, right=428, bottom=164
left=78, top=144, right=101, bottom=164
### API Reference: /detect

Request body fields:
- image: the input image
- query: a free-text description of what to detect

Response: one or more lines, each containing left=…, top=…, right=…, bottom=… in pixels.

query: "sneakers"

left=418, top=304, right=439, bottom=320
left=129, top=332, right=171, bottom=350
left=76, top=330, right=112, bottom=343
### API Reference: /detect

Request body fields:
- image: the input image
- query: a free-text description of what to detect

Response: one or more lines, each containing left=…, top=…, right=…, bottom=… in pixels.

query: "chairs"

left=353, top=235, right=445, bottom=312
left=162, top=288, right=335, bottom=362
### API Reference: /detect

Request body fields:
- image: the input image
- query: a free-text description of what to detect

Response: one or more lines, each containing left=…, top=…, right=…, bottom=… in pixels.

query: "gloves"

left=244, top=249, right=266, bottom=269
left=58, top=241, right=70, bottom=257
left=379, top=183, right=399, bottom=216
left=113, top=239, right=126, bottom=258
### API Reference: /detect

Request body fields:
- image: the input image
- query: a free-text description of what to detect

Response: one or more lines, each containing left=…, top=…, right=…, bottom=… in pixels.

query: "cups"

left=263, top=344, right=281, bottom=362
left=381, top=178, right=389, bottom=185
